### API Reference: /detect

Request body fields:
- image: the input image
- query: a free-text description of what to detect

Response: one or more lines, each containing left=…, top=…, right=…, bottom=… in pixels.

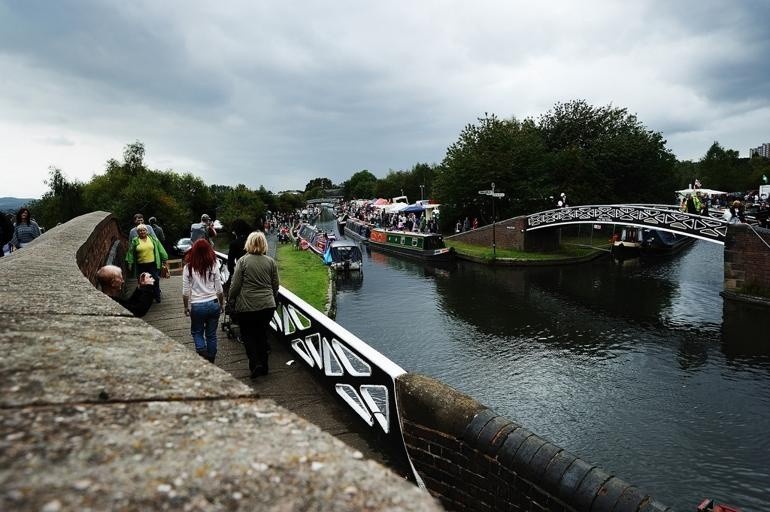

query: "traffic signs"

left=492, top=193, right=505, bottom=197
left=479, top=190, right=493, bottom=195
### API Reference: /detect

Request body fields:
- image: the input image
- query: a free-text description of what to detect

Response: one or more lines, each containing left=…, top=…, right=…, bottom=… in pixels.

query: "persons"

left=1, top=208, right=43, bottom=257
left=224, top=218, right=273, bottom=357
left=455, top=219, right=462, bottom=234
left=125, top=213, right=159, bottom=247
left=95, top=264, right=157, bottom=319
left=147, top=216, right=167, bottom=246
left=472, top=217, right=479, bottom=232
left=262, top=204, right=319, bottom=251
left=224, top=231, right=281, bottom=380
left=180, top=238, right=226, bottom=364
left=678, top=189, right=770, bottom=229
left=122, top=223, right=169, bottom=304
left=560, top=192, right=569, bottom=209
left=334, top=197, right=441, bottom=233
left=190, top=213, right=217, bottom=247
left=463, top=217, right=470, bottom=232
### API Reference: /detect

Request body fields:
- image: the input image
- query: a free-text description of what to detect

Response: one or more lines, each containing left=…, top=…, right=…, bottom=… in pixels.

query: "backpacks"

left=190, top=224, right=206, bottom=244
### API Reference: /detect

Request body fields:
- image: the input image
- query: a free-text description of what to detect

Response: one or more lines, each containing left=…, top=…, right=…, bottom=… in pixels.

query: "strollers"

left=220, top=275, right=243, bottom=340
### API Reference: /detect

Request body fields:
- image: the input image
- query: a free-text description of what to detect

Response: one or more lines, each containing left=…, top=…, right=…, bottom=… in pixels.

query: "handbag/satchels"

left=158, top=265, right=171, bottom=279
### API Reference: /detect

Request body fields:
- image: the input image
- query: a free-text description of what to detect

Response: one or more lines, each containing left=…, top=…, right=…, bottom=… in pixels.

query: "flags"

left=695, top=179, right=702, bottom=188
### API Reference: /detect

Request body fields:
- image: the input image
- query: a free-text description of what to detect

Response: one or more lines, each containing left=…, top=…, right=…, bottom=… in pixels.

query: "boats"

left=334, top=269, right=364, bottom=293
left=295, top=214, right=457, bottom=271
left=612, top=224, right=697, bottom=253
left=613, top=250, right=651, bottom=270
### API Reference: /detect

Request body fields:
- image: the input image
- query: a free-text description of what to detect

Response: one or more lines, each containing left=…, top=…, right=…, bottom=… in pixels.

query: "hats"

left=202, top=213, right=211, bottom=219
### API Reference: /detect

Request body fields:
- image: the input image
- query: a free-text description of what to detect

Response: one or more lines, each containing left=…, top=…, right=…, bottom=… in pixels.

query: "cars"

left=173, top=238, right=193, bottom=256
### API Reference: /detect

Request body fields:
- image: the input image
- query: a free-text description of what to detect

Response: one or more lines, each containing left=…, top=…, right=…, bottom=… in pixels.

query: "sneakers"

left=250, top=360, right=269, bottom=379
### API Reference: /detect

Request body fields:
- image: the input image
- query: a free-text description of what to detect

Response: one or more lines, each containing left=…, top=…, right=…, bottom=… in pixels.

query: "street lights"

left=420, top=185, right=424, bottom=200
